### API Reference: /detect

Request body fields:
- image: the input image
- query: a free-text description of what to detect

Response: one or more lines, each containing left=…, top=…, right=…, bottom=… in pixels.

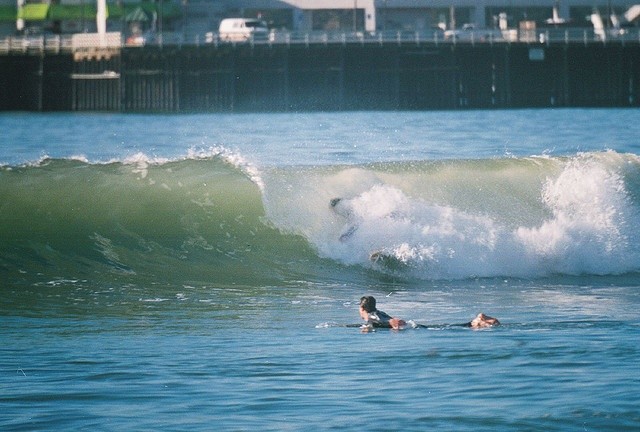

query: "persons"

left=358, top=295, right=500, bottom=331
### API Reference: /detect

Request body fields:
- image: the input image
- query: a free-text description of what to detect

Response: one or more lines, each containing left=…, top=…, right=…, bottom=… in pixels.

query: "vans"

left=217, top=17, right=272, bottom=46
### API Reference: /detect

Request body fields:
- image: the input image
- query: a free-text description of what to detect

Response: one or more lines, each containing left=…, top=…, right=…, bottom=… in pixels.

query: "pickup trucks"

left=443, top=22, right=504, bottom=43
